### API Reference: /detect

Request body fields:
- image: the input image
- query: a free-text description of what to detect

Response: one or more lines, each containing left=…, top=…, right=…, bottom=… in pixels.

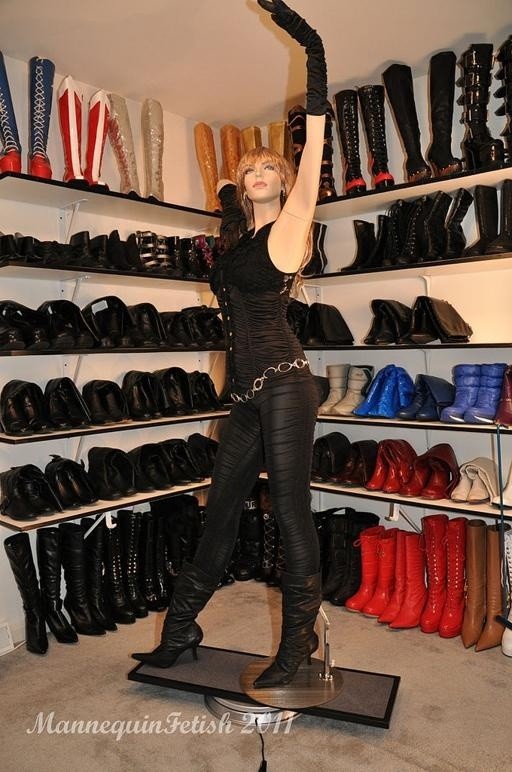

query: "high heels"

left=0, top=231, right=73, bottom=266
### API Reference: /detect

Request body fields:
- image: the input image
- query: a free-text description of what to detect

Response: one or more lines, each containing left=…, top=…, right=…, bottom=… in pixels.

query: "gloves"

left=218, top=183, right=248, bottom=251
left=258, top=0, right=327, bottom=116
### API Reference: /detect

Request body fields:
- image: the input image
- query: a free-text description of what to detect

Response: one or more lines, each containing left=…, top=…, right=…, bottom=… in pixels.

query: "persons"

left=130, top=0, right=329, bottom=690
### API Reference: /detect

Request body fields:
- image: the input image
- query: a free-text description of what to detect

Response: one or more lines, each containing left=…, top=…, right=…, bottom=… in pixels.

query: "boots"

left=194, top=122, right=223, bottom=214
left=252, top=570, right=322, bottom=689
left=342, top=188, right=475, bottom=272
left=428, top=51, right=462, bottom=177
left=335, top=90, right=366, bottom=196
left=301, top=219, right=328, bottom=278
left=483, top=179, right=512, bottom=255
left=455, top=44, right=504, bottom=170
left=287, top=105, right=306, bottom=176
left=285, top=300, right=354, bottom=346
left=106, top=94, right=141, bottom=198
left=28, top=56, right=53, bottom=181
left=0, top=296, right=226, bottom=350
left=0, top=51, right=21, bottom=175
left=493, top=34, right=512, bottom=160
left=57, top=75, right=89, bottom=190
left=140, top=98, right=163, bottom=203
left=220, top=124, right=262, bottom=199
left=0, top=433, right=219, bottom=520
left=314, top=363, right=512, bottom=427
left=131, top=559, right=218, bottom=668
left=463, top=185, right=498, bottom=257
left=311, top=432, right=512, bottom=508
left=311, top=507, right=512, bottom=658
left=70, top=230, right=221, bottom=278
left=0, top=367, right=230, bottom=437
left=318, top=100, right=337, bottom=201
left=410, top=295, right=473, bottom=345
left=268, top=121, right=294, bottom=163
left=364, top=298, right=413, bottom=345
left=382, top=64, right=431, bottom=183
left=84, top=89, right=109, bottom=194
left=358, top=85, right=394, bottom=190
